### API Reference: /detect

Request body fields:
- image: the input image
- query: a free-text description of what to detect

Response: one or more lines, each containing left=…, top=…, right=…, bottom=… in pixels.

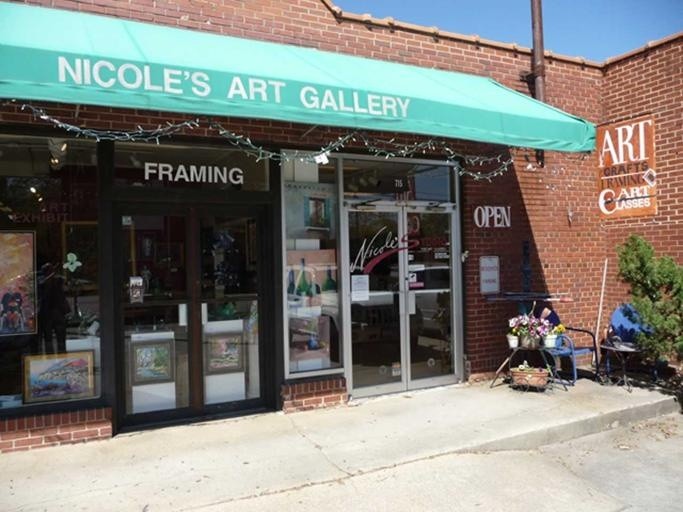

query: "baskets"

left=509, top=367, right=549, bottom=388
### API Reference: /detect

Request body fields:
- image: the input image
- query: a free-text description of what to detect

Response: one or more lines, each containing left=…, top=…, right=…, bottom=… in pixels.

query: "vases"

left=510, top=367, right=548, bottom=391
left=506, top=332, right=561, bottom=349
left=64, top=287, right=84, bottom=324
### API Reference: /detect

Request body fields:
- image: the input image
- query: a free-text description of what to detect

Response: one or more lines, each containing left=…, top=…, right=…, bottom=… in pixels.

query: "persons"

left=31, top=245, right=73, bottom=360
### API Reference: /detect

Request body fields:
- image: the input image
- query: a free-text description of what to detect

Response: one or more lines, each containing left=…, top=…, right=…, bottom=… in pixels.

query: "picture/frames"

left=131, top=331, right=245, bottom=387
left=0, top=228, right=38, bottom=336
left=247, top=219, right=257, bottom=265
left=21, top=349, right=95, bottom=404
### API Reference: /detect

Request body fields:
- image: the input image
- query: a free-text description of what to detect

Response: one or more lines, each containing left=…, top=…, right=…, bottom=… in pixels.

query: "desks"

left=490, top=347, right=569, bottom=393
left=593, top=342, right=647, bottom=394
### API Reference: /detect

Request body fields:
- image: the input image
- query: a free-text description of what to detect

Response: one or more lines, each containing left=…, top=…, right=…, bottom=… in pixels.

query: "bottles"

left=287, top=257, right=337, bottom=308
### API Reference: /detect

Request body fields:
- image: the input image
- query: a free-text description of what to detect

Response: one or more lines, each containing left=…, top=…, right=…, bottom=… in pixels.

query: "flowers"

left=504, top=312, right=567, bottom=335
left=62, top=252, right=85, bottom=313
left=518, top=360, right=545, bottom=372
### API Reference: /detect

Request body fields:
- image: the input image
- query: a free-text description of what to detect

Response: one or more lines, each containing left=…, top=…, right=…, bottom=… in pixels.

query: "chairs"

left=509, top=302, right=599, bottom=394
left=604, top=303, right=658, bottom=382
